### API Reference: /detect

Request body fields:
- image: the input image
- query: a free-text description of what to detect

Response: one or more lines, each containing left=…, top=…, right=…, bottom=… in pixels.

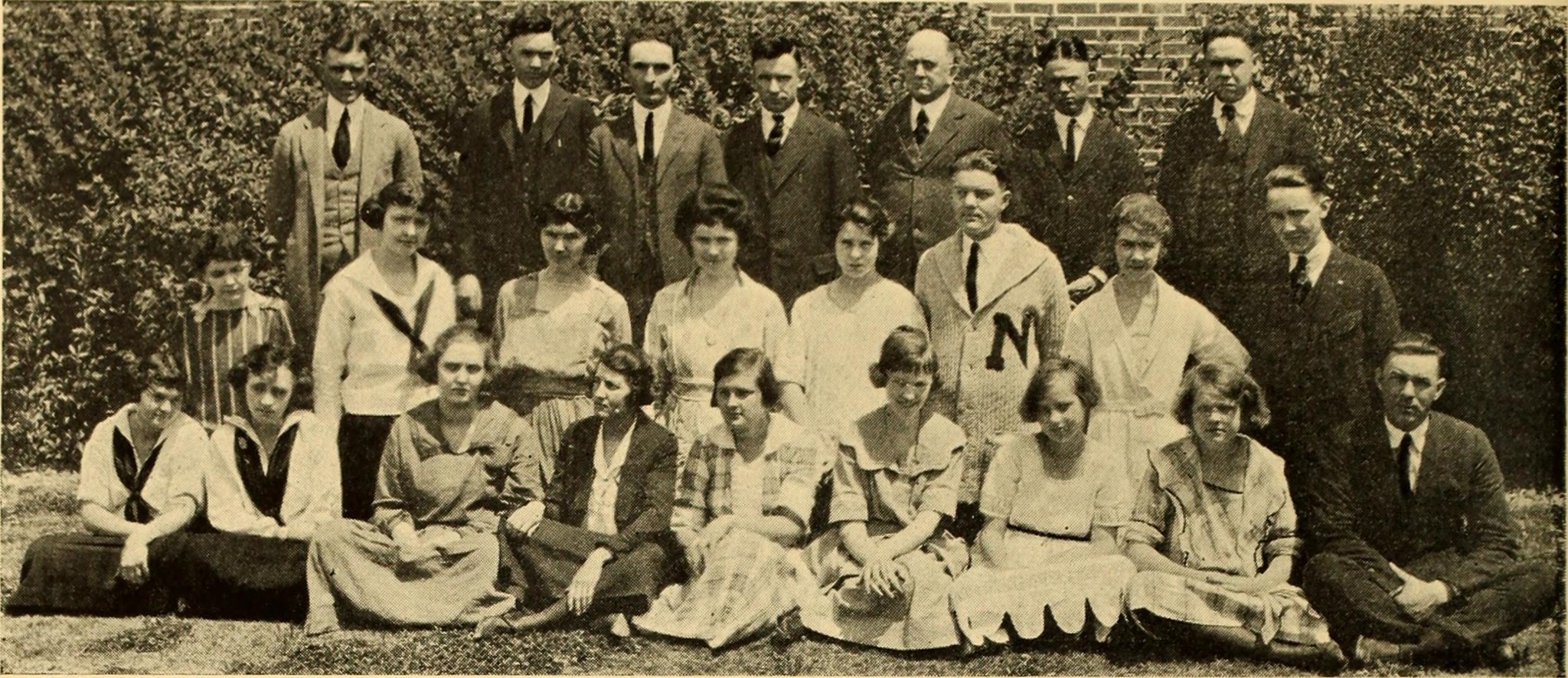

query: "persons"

left=800, top=324, right=970, bottom=651
left=304, top=325, right=542, bottom=638
left=584, top=33, right=726, bottom=347
left=1219, top=165, right=1400, bottom=502
left=474, top=343, right=677, bottom=641
left=452, top=16, right=598, bottom=333
left=725, top=35, right=859, bottom=317
left=311, top=180, right=455, bottom=523
left=1298, top=329, right=1560, bottom=670
left=863, top=28, right=1008, bottom=293
left=788, top=197, right=931, bottom=446
left=157, top=343, right=341, bottom=620
left=267, top=29, right=423, bottom=348
left=1121, top=357, right=1346, bottom=672
left=1060, top=192, right=1252, bottom=500
left=1156, top=26, right=1318, bottom=325
left=915, top=149, right=1072, bottom=542
left=165, top=228, right=299, bottom=434
left=642, top=181, right=789, bottom=496
left=5, top=352, right=208, bottom=615
left=951, top=358, right=1138, bottom=644
left=634, top=349, right=820, bottom=651
left=1004, top=35, right=1148, bottom=309
left=488, top=191, right=635, bottom=487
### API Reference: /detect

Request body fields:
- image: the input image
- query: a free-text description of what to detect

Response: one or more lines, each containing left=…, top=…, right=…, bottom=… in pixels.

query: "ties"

left=964, top=243, right=980, bottom=313
left=1395, top=433, right=1414, bottom=495
left=331, top=107, right=351, bottom=172
left=521, top=94, right=533, bottom=136
left=766, top=114, right=785, bottom=159
left=1222, top=105, right=1245, bottom=160
left=642, top=112, right=653, bottom=167
left=912, top=108, right=931, bottom=146
left=1066, top=119, right=1078, bottom=170
left=1288, top=255, right=1312, bottom=306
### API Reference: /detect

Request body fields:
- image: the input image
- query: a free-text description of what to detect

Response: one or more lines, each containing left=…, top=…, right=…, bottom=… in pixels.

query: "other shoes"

left=1452, top=642, right=1516, bottom=670
left=961, top=630, right=997, bottom=658
left=1348, top=633, right=1398, bottom=668
left=590, top=611, right=631, bottom=639
left=472, top=613, right=519, bottom=640
left=1308, top=636, right=1346, bottom=675
left=775, top=611, right=809, bottom=643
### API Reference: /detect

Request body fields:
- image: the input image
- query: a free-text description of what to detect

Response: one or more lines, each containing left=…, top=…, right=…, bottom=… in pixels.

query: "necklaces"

left=694, top=270, right=738, bottom=346
